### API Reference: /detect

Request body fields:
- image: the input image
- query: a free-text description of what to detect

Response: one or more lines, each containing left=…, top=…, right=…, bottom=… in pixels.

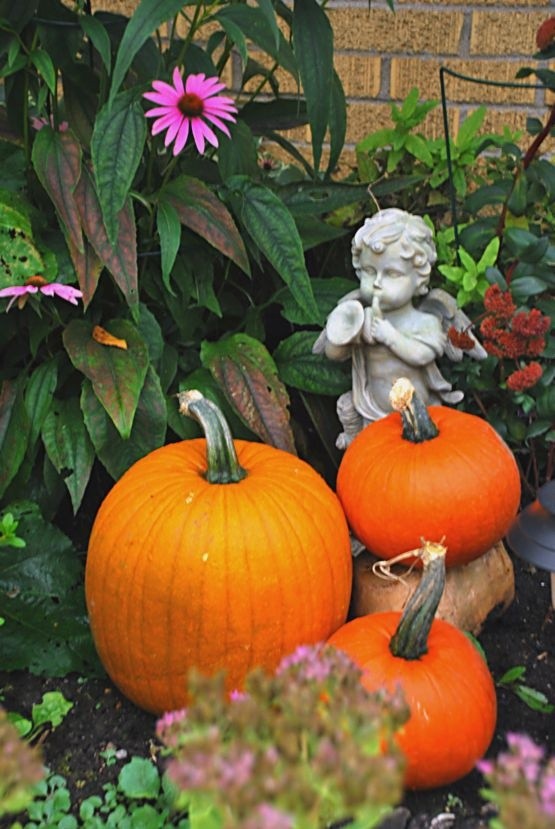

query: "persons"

left=312, top=208, right=487, bottom=448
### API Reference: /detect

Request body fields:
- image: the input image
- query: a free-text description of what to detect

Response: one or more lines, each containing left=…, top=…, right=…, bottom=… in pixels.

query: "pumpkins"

left=336, top=378, right=521, bottom=569
left=82, top=388, right=352, bottom=715
left=308, top=540, right=497, bottom=790
